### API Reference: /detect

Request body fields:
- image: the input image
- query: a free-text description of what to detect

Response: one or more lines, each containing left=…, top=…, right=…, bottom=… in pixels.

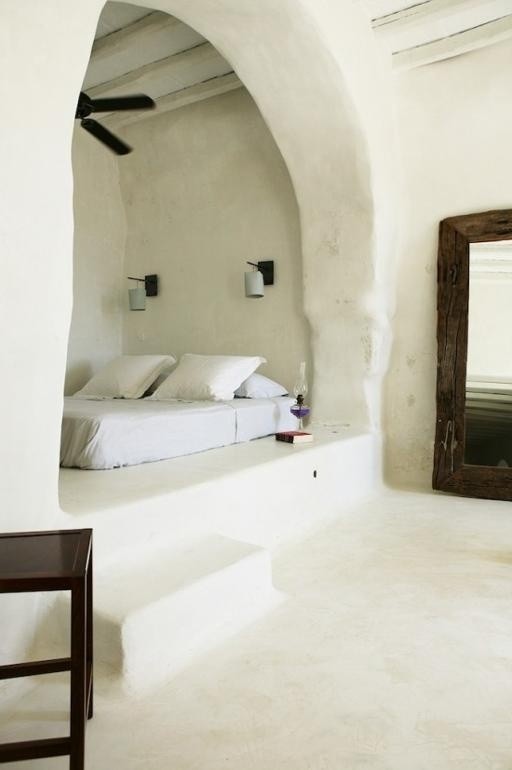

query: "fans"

left=73, top=92, right=155, bottom=155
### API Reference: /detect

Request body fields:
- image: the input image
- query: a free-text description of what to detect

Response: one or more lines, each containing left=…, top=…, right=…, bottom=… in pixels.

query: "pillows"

left=234, top=372, right=288, bottom=400
left=145, top=353, right=267, bottom=402
left=73, top=353, right=177, bottom=400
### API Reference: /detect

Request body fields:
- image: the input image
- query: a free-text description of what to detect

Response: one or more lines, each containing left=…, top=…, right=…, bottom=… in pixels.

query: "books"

left=276, top=430, right=313, bottom=445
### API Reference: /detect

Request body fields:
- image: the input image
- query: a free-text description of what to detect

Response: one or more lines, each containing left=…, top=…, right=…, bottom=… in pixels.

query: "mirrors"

left=431, top=209, right=512, bottom=505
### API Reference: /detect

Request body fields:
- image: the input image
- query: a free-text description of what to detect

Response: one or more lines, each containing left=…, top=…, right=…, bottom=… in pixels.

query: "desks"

left=0, top=528, right=93, bottom=770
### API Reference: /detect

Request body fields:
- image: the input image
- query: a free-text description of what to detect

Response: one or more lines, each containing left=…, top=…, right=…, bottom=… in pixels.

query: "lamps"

left=244, top=261, right=274, bottom=298
left=127, top=274, right=157, bottom=311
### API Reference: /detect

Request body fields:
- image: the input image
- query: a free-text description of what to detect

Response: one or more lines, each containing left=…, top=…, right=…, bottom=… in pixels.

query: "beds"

left=62, top=392, right=301, bottom=469
